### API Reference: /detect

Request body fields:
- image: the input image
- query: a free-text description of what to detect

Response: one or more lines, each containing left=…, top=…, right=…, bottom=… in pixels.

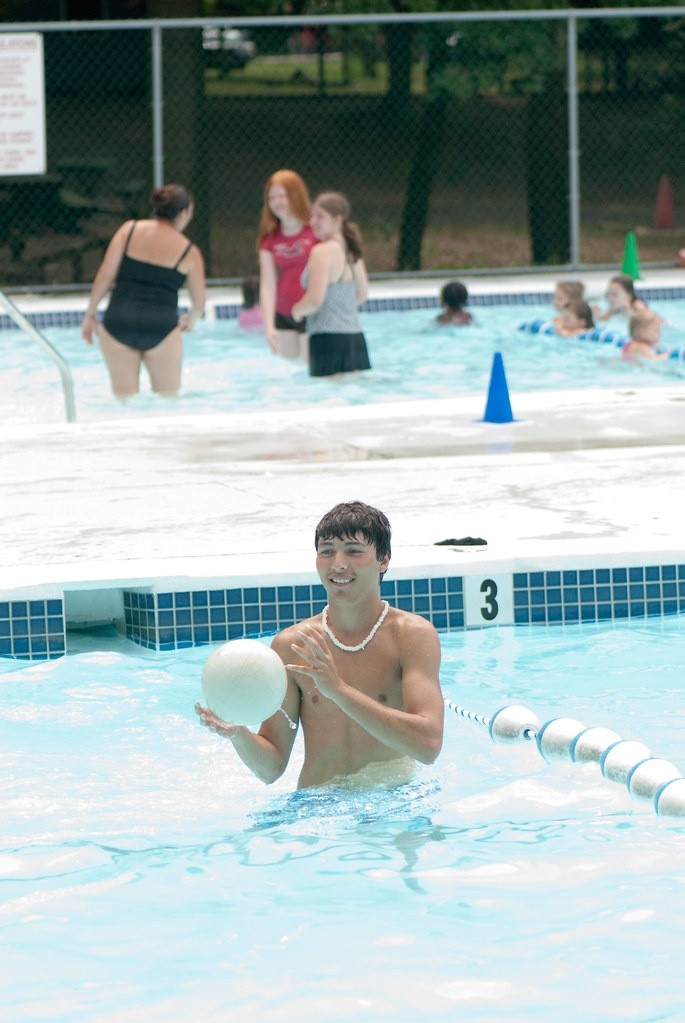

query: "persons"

left=256, top=170, right=321, bottom=362
left=555, top=280, right=594, bottom=335
left=593, top=275, right=665, bottom=324
left=239, top=278, right=262, bottom=330
left=291, top=194, right=371, bottom=377
left=82, top=185, right=205, bottom=396
left=437, top=282, right=472, bottom=324
left=623, top=310, right=668, bottom=359
left=195, top=500, right=445, bottom=791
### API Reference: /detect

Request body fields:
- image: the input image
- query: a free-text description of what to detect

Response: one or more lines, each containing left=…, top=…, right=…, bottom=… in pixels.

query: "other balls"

left=201, top=638, right=288, bottom=726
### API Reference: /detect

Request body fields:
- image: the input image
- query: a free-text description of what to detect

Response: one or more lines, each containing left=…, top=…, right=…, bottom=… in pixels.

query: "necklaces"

left=323, top=600, right=389, bottom=652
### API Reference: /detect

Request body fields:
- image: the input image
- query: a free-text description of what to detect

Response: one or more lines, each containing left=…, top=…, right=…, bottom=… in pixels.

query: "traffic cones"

left=482, top=351, right=513, bottom=423
left=651, top=175, right=675, bottom=230
left=621, top=231, right=641, bottom=281
